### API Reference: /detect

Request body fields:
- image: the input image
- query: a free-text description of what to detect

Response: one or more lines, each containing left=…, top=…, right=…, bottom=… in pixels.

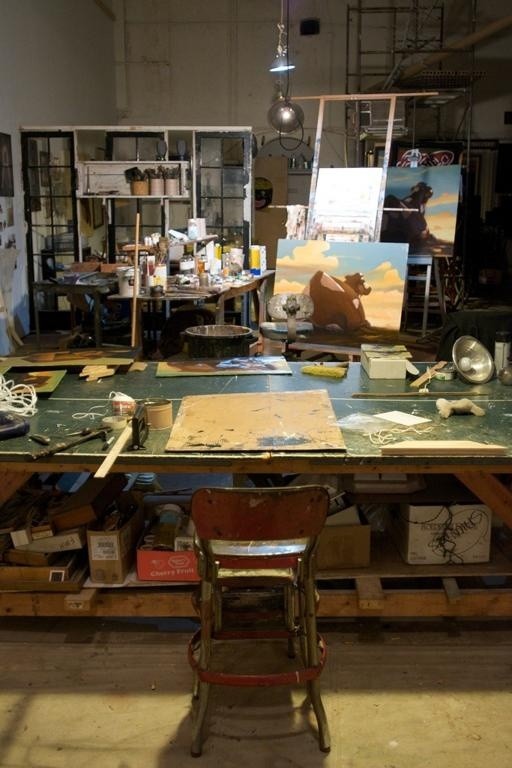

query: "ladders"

left=403, top=255, right=446, bottom=337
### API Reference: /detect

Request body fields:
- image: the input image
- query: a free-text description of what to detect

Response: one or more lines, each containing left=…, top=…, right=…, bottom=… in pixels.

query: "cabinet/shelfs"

left=20, top=124, right=253, bottom=265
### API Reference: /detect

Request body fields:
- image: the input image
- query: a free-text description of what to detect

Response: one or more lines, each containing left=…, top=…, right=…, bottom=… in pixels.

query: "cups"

left=149, top=178, right=166, bottom=196
left=165, top=178, right=181, bottom=197
left=131, top=180, right=150, bottom=195
left=112, top=401, right=136, bottom=421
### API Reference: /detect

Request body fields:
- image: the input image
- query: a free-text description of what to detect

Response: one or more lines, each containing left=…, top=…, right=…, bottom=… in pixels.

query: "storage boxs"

left=319, top=519, right=371, bottom=572
left=87, top=516, right=133, bottom=585
left=396, top=491, right=492, bottom=566
left=135, top=523, right=202, bottom=583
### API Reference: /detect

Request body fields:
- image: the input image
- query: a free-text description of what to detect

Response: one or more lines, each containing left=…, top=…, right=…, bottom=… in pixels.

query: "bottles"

left=152, top=502, right=184, bottom=551
left=495, top=331, right=511, bottom=380
left=287, top=153, right=314, bottom=169
left=153, top=245, right=234, bottom=292
left=367, top=150, right=375, bottom=168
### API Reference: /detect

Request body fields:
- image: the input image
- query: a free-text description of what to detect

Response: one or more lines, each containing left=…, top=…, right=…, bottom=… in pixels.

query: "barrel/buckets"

left=117, top=267, right=139, bottom=296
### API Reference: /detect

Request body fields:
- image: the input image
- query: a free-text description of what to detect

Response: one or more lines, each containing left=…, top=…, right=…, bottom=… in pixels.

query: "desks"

left=0, top=347, right=512, bottom=625
left=34, top=266, right=276, bottom=346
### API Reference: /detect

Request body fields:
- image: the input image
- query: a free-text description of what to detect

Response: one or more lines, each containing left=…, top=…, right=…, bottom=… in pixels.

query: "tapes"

left=102, top=415, right=126, bottom=429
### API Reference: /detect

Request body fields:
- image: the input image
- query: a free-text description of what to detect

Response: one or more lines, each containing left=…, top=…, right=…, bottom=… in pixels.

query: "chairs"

left=180, top=485, right=332, bottom=758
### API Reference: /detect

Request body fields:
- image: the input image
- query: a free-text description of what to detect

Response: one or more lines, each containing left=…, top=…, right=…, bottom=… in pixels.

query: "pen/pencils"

left=102, top=436, right=115, bottom=450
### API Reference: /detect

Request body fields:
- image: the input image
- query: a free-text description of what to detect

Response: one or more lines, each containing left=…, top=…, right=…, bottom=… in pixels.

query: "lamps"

left=440, top=333, right=495, bottom=385
left=269, top=0, right=295, bottom=74
left=268, top=0, right=307, bottom=152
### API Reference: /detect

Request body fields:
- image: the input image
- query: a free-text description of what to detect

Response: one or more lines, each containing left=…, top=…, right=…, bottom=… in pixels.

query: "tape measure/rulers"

left=409, top=360, right=448, bottom=389
